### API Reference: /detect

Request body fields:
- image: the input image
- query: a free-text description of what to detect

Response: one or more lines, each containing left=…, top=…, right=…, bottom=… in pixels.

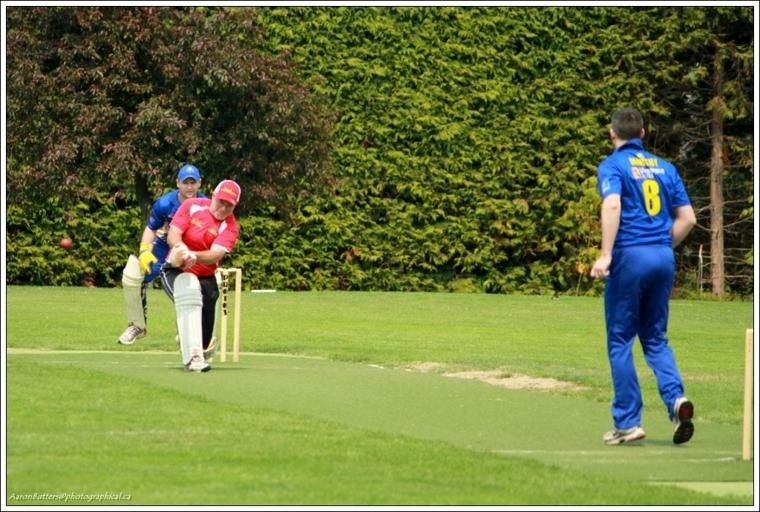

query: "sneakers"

left=673, top=396, right=694, bottom=444
left=118, top=324, right=147, bottom=345
left=184, top=356, right=211, bottom=372
left=604, top=427, right=646, bottom=446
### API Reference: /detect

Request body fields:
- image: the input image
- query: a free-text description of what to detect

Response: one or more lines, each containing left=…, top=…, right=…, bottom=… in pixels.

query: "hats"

left=213, top=180, right=241, bottom=207
left=178, top=164, right=201, bottom=184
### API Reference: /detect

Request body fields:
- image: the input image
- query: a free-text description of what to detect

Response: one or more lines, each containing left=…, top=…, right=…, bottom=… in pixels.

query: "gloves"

left=165, top=243, right=197, bottom=270
left=139, top=241, right=158, bottom=276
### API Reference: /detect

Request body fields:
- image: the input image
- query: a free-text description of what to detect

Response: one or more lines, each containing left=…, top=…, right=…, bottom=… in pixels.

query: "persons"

left=590, top=108, right=698, bottom=446
left=118, top=164, right=207, bottom=345
left=159, top=179, right=242, bottom=372
